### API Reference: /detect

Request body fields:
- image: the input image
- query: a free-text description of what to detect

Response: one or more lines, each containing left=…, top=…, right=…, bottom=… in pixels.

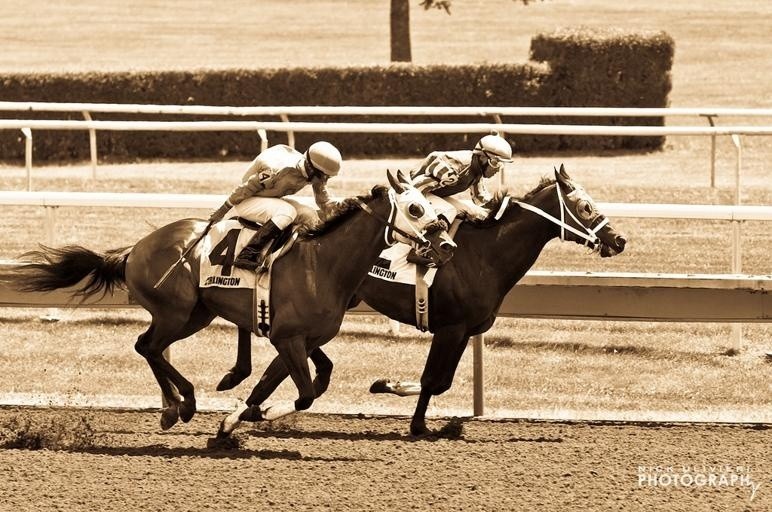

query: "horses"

left=217, top=162, right=628, bottom=438
left=0, top=168, right=458, bottom=433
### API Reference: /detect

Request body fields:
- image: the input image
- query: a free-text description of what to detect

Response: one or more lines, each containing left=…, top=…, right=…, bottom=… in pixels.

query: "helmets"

left=472, top=135, right=515, bottom=162
left=308, top=141, right=343, bottom=175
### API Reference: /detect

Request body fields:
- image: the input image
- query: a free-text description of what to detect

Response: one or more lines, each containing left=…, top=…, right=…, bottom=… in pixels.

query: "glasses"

left=488, top=156, right=504, bottom=169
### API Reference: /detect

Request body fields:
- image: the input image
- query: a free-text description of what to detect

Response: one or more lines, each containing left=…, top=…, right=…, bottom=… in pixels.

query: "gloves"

left=208, top=199, right=232, bottom=225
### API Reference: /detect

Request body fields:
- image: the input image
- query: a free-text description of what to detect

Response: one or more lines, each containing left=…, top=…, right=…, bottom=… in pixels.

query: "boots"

left=234, top=219, right=282, bottom=273
left=406, top=214, right=451, bottom=268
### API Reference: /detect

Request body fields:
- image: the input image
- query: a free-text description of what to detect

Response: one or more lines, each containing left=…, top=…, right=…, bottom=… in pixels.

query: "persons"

left=209, top=141, right=342, bottom=269
left=407, top=135, right=514, bottom=266
